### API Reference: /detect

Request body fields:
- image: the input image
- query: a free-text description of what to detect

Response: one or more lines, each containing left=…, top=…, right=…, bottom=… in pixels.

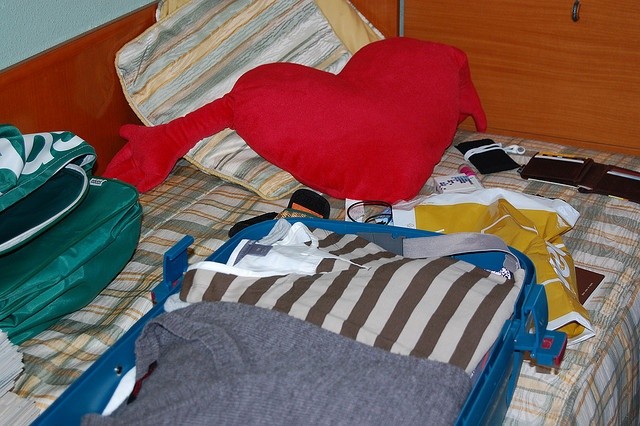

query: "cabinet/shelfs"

left=349, top=0, right=640, bottom=156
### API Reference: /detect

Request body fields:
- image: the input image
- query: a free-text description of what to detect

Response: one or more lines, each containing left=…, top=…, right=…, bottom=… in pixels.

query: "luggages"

left=29, top=218, right=567, bottom=426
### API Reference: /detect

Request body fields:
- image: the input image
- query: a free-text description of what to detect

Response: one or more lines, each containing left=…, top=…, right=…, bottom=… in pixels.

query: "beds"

left=1, top=1, right=640, bottom=425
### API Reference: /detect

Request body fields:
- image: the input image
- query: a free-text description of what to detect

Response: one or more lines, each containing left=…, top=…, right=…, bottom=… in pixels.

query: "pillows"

left=112, top=9, right=387, bottom=202
left=102, top=34, right=488, bottom=207
left=155, top=0, right=385, bottom=56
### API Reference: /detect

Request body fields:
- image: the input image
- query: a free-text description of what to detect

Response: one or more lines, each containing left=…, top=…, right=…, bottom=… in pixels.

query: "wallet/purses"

left=517, top=150, right=640, bottom=205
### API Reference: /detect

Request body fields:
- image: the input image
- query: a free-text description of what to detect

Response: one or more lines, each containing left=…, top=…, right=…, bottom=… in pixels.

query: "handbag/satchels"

left=392, top=187, right=596, bottom=348
left=0, top=125, right=143, bottom=343
